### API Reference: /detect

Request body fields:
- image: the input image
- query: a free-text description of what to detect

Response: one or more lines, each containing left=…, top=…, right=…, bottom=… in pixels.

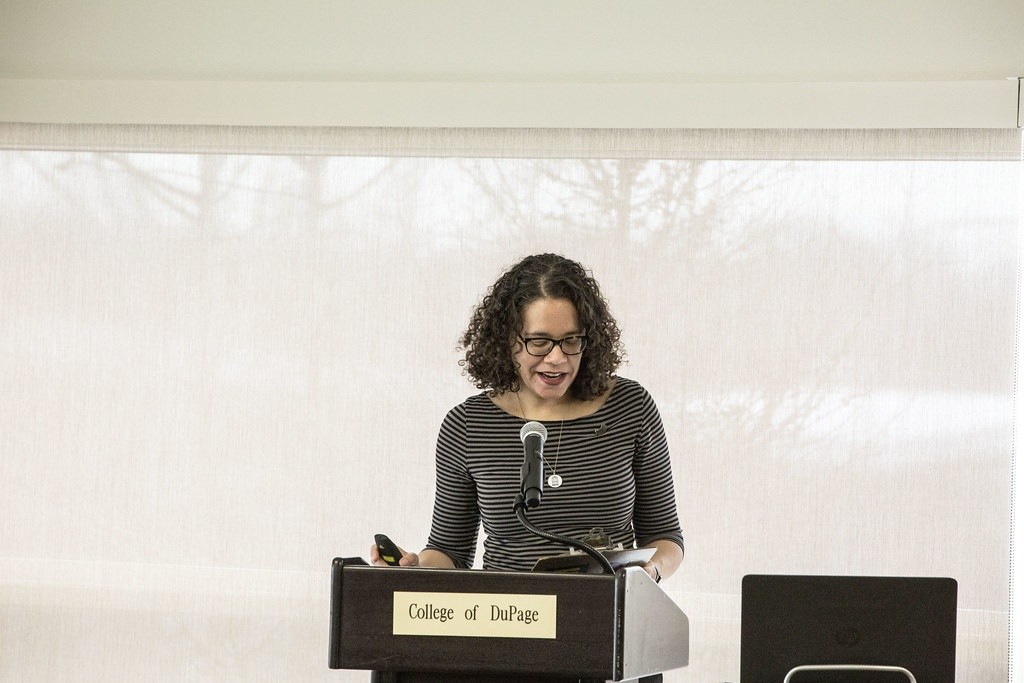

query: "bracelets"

left=654, top=566, right=664, bottom=586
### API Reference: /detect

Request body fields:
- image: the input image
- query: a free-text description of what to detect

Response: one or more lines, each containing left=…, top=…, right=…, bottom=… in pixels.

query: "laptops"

left=739, top=574, right=957, bottom=683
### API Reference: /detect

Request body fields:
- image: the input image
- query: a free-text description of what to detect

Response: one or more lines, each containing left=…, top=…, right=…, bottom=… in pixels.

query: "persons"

left=369, top=252, right=685, bottom=681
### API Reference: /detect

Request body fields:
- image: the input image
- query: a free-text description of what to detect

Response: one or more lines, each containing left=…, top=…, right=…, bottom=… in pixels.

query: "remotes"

left=374, top=534, right=403, bottom=566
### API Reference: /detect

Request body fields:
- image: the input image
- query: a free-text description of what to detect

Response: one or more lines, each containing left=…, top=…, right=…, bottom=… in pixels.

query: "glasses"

left=516, top=329, right=589, bottom=356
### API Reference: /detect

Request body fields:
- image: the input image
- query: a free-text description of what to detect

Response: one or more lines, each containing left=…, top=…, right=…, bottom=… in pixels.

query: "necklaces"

left=511, top=382, right=570, bottom=489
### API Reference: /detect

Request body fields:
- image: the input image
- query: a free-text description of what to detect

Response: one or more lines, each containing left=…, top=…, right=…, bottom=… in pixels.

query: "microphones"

left=520, top=421, right=547, bottom=507
